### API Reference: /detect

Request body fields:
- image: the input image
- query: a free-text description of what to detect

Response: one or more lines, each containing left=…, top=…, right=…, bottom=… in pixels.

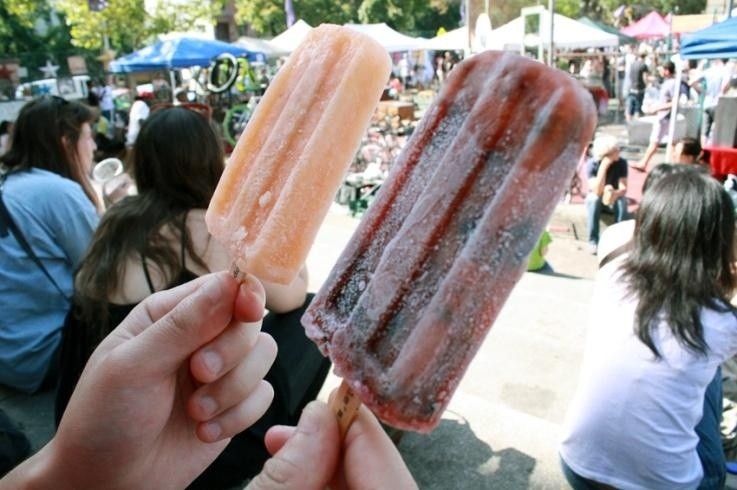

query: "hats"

left=662, top=60, right=674, bottom=73
left=136, top=83, right=156, bottom=99
left=593, top=131, right=618, bottom=161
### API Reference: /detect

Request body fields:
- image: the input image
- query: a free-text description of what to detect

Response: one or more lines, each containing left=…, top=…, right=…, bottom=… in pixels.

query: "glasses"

left=149, top=103, right=213, bottom=127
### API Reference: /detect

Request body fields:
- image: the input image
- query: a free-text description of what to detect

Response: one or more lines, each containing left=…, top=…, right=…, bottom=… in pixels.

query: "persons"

left=56, top=105, right=332, bottom=490
left=0, top=95, right=107, bottom=396
left=0, top=121, right=13, bottom=156
left=86, top=48, right=737, bottom=272
left=0, top=271, right=419, bottom=490
left=558, top=171, right=737, bottom=490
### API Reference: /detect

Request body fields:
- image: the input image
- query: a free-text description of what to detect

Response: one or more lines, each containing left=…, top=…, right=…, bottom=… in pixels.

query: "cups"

left=93, top=157, right=123, bottom=198
